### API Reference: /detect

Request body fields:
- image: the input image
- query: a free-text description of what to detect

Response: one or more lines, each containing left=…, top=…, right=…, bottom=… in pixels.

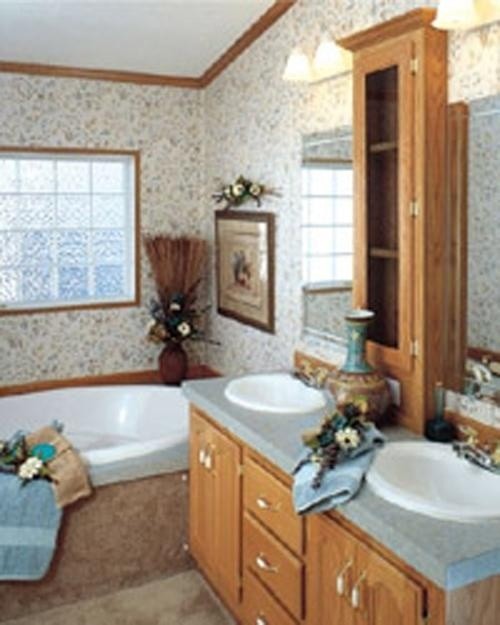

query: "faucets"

left=291, top=361, right=327, bottom=390
left=453, top=424, right=500, bottom=473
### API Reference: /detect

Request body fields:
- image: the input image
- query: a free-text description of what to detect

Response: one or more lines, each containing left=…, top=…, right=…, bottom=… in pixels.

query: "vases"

left=159, top=340, right=187, bottom=386
left=324, top=308, right=392, bottom=426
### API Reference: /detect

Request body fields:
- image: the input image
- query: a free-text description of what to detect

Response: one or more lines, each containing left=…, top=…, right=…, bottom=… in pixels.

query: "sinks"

left=364, top=440, right=500, bottom=524
left=224, top=373, right=326, bottom=415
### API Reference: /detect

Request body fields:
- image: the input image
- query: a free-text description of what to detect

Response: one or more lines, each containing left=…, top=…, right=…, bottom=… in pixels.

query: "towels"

left=291, top=421, right=382, bottom=515
left=0, top=420, right=94, bottom=583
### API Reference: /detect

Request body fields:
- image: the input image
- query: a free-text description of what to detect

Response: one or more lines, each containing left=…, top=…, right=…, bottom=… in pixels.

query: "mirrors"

left=299, top=127, right=356, bottom=348
left=445, top=85, right=499, bottom=409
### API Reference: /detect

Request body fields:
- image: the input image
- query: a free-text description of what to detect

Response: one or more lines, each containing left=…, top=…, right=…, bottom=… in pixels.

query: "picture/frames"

left=214, top=209, right=275, bottom=335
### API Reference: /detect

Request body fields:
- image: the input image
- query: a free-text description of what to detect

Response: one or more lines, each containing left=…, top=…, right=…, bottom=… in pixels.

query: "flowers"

left=221, top=180, right=263, bottom=205
left=146, top=280, right=220, bottom=350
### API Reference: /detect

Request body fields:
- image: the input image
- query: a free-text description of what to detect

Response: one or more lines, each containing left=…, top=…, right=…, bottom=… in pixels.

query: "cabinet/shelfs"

left=189, top=401, right=240, bottom=625
left=303, top=507, right=446, bottom=625
left=241, top=450, right=303, bottom=624
left=337, top=8, right=446, bottom=434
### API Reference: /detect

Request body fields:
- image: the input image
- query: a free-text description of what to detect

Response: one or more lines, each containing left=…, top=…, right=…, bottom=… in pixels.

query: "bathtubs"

left=0, top=385, right=190, bottom=464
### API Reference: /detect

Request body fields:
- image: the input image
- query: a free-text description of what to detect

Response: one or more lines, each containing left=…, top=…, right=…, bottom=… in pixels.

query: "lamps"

left=280, top=28, right=350, bottom=86
left=434, top=3, right=499, bottom=32
